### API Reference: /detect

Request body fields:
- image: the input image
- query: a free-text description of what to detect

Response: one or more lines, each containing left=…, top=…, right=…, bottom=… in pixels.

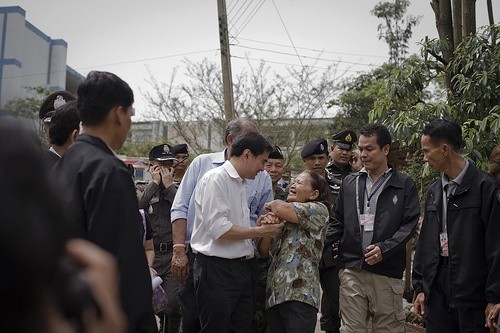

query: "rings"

left=375, top=254, right=378, bottom=258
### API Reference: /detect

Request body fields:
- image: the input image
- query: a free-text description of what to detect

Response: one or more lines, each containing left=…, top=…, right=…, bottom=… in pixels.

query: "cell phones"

left=149, top=162, right=158, bottom=170
left=351, top=155, right=354, bottom=163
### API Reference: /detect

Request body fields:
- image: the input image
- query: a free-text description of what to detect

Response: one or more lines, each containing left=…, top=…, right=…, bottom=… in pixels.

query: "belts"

left=154, top=242, right=173, bottom=253
left=198, top=252, right=256, bottom=264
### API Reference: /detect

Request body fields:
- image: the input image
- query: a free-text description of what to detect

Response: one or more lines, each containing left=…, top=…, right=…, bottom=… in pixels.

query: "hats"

left=39, top=91, right=76, bottom=124
left=149, top=143, right=177, bottom=161
left=268, top=146, right=284, bottom=159
left=331, top=130, right=357, bottom=149
left=301, top=139, right=327, bottom=158
left=173, top=144, right=189, bottom=155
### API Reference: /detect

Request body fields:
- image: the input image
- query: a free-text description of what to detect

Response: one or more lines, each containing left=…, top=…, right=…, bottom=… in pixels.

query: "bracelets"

left=173, top=244, right=186, bottom=248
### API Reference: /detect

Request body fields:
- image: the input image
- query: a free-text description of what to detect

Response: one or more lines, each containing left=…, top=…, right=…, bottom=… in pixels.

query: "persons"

left=263, top=145, right=287, bottom=202
left=322, top=122, right=421, bottom=333
left=257, top=169, right=332, bottom=333
left=408, top=118, right=500, bottom=333
left=488, top=144, right=500, bottom=181
left=51, top=69, right=160, bottom=333
left=47, top=99, right=85, bottom=165
left=0, top=116, right=129, bottom=333
left=325, top=129, right=363, bottom=181
left=138, top=143, right=189, bottom=333
left=190, top=129, right=285, bottom=333
left=488, top=304, right=500, bottom=333
left=170, top=116, right=274, bottom=333
left=38, top=91, right=78, bottom=142
left=300, top=138, right=342, bottom=333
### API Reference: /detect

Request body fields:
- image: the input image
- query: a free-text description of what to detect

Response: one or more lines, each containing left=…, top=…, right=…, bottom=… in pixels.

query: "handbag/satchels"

left=149, top=267, right=168, bottom=314
